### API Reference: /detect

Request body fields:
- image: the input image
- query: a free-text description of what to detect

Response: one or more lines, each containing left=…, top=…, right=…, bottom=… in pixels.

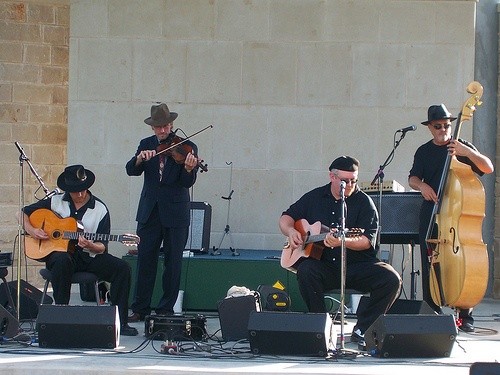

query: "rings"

left=453, top=148, right=455, bottom=151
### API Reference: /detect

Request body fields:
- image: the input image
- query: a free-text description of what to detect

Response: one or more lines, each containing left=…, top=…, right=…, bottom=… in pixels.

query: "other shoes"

left=351, top=331, right=367, bottom=351
left=459, top=323, right=474, bottom=332
left=128, top=312, right=145, bottom=322
left=120, top=323, right=138, bottom=336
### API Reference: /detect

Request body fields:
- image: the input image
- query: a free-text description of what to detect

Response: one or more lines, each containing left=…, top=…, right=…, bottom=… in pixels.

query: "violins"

left=155, top=133, right=209, bottom=174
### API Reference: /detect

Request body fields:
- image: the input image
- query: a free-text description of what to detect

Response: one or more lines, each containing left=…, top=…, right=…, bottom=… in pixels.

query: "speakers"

left=184, top=202, right=212, bottom=253
left=217, top=290, right=336, bottom=357
left=364, top=192, right=423, bottom=245
left=0, top=279, right=120, bottom=349
left=356, top=296, right=456, bottom=359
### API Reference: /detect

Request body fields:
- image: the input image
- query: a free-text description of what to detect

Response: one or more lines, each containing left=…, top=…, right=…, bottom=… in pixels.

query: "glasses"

left=334, top=173, right=359, bottom=184
left=429, top=123, right=452, bottom=130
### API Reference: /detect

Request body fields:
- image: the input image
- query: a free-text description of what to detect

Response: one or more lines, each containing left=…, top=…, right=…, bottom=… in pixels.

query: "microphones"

left=397, top=125, right=417, bottom=132
left=339, top=181, right=347, bottom=188
left=41, top=188, right=60, bottom=200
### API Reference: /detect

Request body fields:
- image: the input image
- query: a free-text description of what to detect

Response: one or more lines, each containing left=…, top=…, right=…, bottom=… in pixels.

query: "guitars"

left=280, top=218, right=364, bottom=274
left=23, top=208, right=141, bottom=263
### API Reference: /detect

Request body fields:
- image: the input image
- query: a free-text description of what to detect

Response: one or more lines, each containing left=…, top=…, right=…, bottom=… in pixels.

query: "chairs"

left=325, top=289, right=366, bottom=322
left=37, top=269, right=105, bottom=305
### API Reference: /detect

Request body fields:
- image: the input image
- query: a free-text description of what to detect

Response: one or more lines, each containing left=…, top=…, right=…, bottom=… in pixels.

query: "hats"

left=57, top=165, right=95, bottom=193
left=144, top=102, right=178, bottom=127
left=328, top=156, right=360, bottom=172
left=421, top=104, right=457, bottom=126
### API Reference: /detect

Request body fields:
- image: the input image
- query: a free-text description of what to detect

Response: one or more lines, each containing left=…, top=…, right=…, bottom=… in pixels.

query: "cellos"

left=424, top=79, right=490, bottom=335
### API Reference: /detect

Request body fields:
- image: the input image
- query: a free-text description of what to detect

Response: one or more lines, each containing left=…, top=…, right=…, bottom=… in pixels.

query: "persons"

left=279, top=155, right=403, bottom=342
left=128, top=102, right=198, bottom=323
left=15, top=165, right=138, bottom=336
left=409, top=104, right=494, bottom=332
